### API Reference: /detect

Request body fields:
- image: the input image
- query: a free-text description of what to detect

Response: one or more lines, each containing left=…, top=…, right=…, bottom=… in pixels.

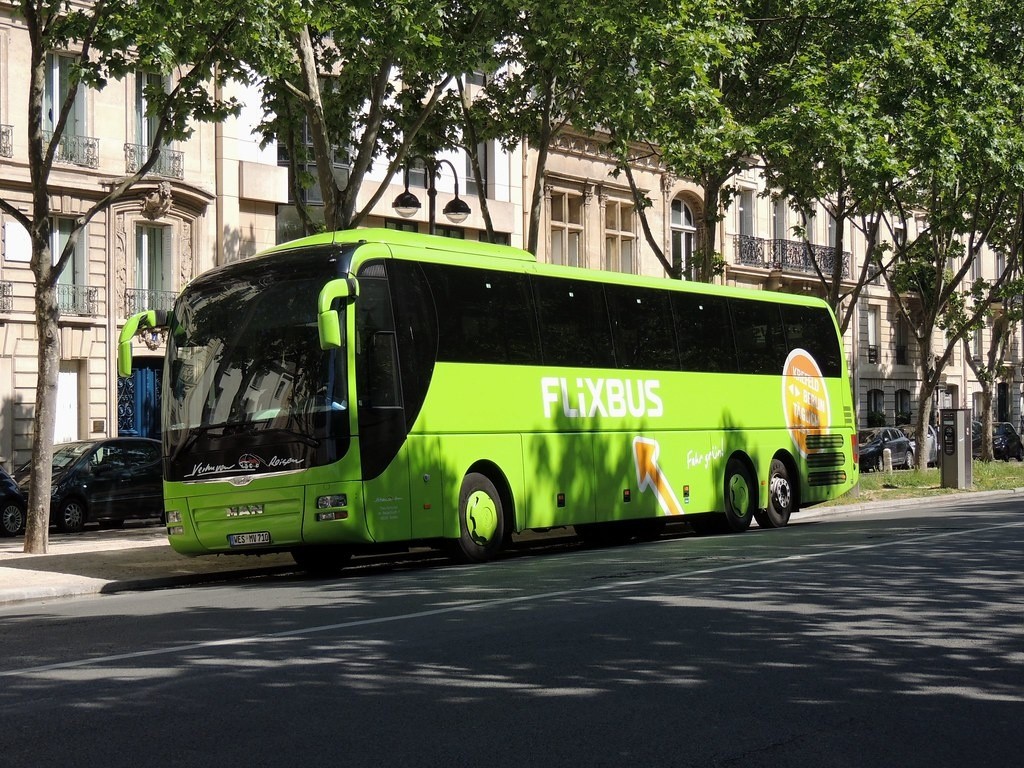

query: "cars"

left=992, top=421, right=1024, bottom=462
left=859, top=427, right=914, bottom=472
left=970, top=421, right=983, bottom=459
left=0, top=464, right=28, bottom=538
left=895, top=424, right=938, bottom=467
left=11, top=437, right=167, bottom=533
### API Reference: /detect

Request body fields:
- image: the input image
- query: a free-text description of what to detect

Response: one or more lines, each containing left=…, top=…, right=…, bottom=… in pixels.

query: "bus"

left=117, top=228, right=860, bottom=582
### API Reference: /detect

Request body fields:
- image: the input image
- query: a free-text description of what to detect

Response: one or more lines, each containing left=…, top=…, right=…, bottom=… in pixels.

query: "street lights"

left=392, top=154, right=472, bottom=235
